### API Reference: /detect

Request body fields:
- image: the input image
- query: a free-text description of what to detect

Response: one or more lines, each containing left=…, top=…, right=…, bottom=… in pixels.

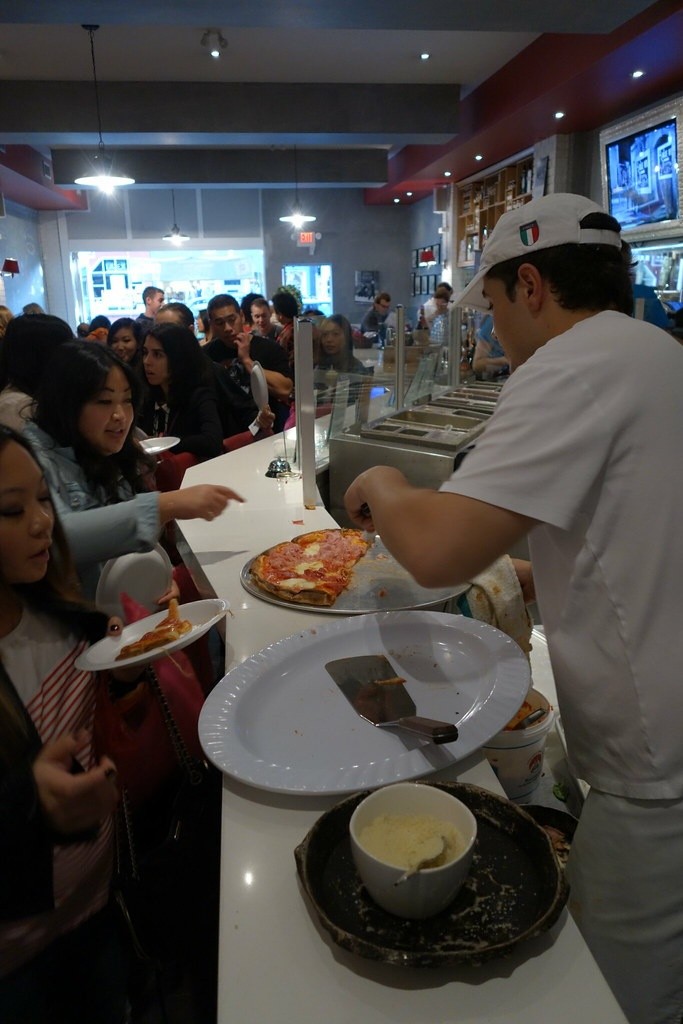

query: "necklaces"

left=145, top=664, right=200, bottom=784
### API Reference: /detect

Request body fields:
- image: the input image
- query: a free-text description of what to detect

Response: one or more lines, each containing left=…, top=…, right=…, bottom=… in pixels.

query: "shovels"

left=324, top=654, right=459, bottom=745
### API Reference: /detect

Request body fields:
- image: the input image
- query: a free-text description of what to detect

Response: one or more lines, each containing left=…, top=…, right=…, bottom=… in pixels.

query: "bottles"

left=412, top=303, right=431, bottom=346
left=325, top=366, right=337, bottom=389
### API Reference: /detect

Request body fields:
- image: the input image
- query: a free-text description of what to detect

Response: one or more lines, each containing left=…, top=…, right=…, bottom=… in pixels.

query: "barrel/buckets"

left=483, top=686, right=554, bottom=799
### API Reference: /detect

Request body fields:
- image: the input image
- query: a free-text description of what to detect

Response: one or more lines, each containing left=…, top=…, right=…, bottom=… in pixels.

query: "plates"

left=138, top=437, right=181, bottom=455
left=251, top=361, right=268, bottom=411
left=95, top=544, right=173, bottom=627
left=74, top=599, right=230, bottom=671
left=198, top=611, right=531, bottom=796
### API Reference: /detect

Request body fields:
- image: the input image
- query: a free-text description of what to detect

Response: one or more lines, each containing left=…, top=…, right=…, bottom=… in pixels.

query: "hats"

left=451, top=194, right=624, bottom=314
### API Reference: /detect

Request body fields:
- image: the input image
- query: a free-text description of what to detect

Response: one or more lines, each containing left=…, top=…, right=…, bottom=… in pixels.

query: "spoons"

left=392, top=836, right=446, bottom=886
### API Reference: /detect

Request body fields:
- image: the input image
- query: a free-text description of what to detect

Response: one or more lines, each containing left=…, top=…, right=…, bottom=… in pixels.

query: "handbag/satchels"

left=91, top=649, right=210, bottom=800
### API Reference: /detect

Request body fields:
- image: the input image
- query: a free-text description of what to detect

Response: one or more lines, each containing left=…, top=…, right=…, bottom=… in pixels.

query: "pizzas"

left=249, top=528, right=369, bottom=607
left=113, top=596, right=192, bottom=662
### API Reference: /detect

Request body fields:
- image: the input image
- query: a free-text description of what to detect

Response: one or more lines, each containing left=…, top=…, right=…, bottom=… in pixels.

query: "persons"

left=78, top=286, right=223, bottom=452
left=0, top=304, right=247, bottom=1024
left=417, top=282, right=453, bottom=328
left=620, top=238, right=673, bottom=335
left=359, top=292, right=393, bottom=334
left=197, top=283, right=371, bottom=433
left=473, top=315, right=509, bottom=379
left=352, top=193, right=683, bottom=1024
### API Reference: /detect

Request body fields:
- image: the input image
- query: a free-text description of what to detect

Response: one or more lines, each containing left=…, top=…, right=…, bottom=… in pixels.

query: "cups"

left=348, top=784, right=477, bottom=921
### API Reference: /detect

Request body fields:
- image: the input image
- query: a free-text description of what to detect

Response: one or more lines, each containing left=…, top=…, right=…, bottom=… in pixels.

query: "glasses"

left=377, top=302, right=391, bottom=311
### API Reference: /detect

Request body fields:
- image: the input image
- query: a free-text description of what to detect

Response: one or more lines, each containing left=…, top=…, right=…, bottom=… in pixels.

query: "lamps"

left=200, top=27, right=228, bottom=49
left=420, top=249, right=446, bottom=269
left=278, top=145, right=317, bottom=228
left=0, top=257, right=20, bottom=279
left=74, top=24, right=136, bottom=195
left=162, top=190, right=190, bottom=245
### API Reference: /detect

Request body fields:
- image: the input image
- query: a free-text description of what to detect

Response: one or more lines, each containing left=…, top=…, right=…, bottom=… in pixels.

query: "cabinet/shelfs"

left=456, top=154, right=534, bottom=269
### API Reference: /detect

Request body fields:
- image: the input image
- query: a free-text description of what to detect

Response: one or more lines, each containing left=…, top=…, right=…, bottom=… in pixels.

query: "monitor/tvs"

left=603, top=114, right=678, bottom=232
